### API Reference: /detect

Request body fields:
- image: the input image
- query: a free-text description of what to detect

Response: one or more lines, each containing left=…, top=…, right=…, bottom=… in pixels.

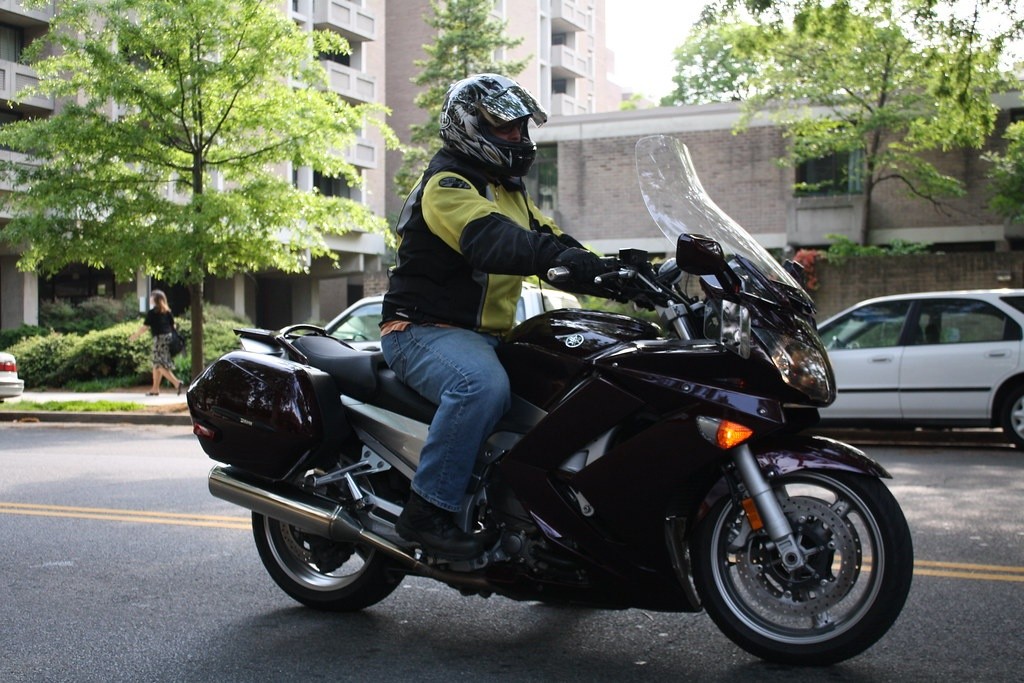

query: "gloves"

left=540, top=244, right=631, bottom=304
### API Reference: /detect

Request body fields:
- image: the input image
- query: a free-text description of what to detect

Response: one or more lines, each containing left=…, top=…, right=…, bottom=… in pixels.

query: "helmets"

left=439, top=78, right=545, bottom=177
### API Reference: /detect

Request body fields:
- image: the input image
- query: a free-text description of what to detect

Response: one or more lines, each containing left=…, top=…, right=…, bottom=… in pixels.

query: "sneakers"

left=396, top=500, right=484, bottom=562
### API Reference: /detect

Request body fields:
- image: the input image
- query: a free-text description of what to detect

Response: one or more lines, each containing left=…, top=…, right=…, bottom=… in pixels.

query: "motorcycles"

left=183, top=133, right=918, bottom=670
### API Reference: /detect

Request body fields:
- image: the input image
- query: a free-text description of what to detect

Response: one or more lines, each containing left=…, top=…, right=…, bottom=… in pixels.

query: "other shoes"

left=147, top=391, right=159, bottom=395
left=176, top=381, right=183, bottom=395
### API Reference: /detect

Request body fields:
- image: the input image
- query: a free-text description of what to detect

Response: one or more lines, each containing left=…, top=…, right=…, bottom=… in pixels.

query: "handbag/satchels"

left=168, top=329, right=185, bottom=357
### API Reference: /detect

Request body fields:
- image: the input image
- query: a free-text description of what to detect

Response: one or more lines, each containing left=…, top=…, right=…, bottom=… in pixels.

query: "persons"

left=376, top=72, right=670, bottom=564
left=127, top=290, right=184, bottom=395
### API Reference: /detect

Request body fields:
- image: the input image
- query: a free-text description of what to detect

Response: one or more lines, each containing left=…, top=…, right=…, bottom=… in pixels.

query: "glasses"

left=494, top=118, right=521, bottom=134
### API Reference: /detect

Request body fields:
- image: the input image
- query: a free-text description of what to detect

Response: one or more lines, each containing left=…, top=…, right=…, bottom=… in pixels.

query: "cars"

left=0, top=350, right=25, bottom=404
left=811, top=288, right=1024, bottom=450
left=315, top=278, right=584, bottom=355
